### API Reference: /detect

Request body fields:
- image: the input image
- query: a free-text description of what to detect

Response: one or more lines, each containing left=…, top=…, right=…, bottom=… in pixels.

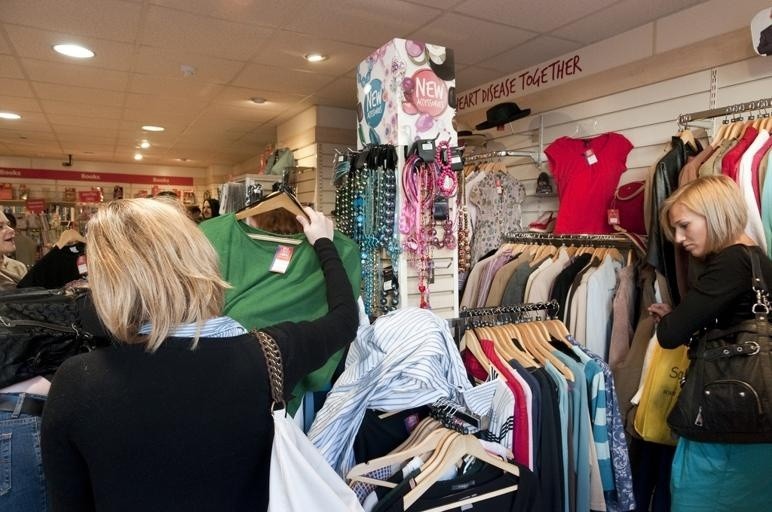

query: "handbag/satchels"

left=0, top=285, right=97, bottom=389
left=609, top=180, right=648, bottom=235
left=633, top=341, right=692, bottom=445
left=268, top=400, right=366, bottom=512
left=666, top=313, right=772, bottom=445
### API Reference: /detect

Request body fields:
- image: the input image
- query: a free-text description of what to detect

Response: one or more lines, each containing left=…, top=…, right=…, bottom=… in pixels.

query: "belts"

left=0, top=394, right=46, bottom=416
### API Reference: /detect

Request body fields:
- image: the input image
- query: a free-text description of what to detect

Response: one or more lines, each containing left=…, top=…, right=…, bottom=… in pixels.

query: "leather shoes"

left=536, top=173, right=552, bottom=195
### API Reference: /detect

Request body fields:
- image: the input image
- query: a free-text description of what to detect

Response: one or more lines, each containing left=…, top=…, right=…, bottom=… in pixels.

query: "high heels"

left=528, top=211, right=557, bottom=233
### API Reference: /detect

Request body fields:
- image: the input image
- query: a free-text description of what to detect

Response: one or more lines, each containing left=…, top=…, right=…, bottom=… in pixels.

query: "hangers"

left=345, top=397, right=536, bottom=512
left=461, top=303, right=617, bottom=405
left=471, top=232, right=632, bottom=301
left=195, top=172, right=360, bottom=253
left=32, top=221, right=90, bottom=263
left=650, top=100, right=771, bottom=179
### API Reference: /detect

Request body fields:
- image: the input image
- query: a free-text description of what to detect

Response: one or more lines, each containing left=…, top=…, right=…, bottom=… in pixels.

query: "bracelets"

left=437, top=170, right=457, bottom=196
left=436, top=140, right=452, bottom=170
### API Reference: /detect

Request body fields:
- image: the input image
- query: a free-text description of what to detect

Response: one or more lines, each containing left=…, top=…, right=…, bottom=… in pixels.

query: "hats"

left=476, top=102, right=531, bottom=130
left=456, top=123, right=486, bottom=143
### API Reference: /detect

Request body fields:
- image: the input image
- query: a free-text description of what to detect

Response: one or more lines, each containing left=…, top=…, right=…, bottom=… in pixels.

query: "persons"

left=38, top=194, right=361, bottom=511
left=0, top=205, right=30, bottom=291
left=201, top=198, right=220, bottom=220
left=0, top=285, right=112, bottom=511
left=191, top=206, right=202, bottom=224
left=645, top=174, right=771, bottom=512
